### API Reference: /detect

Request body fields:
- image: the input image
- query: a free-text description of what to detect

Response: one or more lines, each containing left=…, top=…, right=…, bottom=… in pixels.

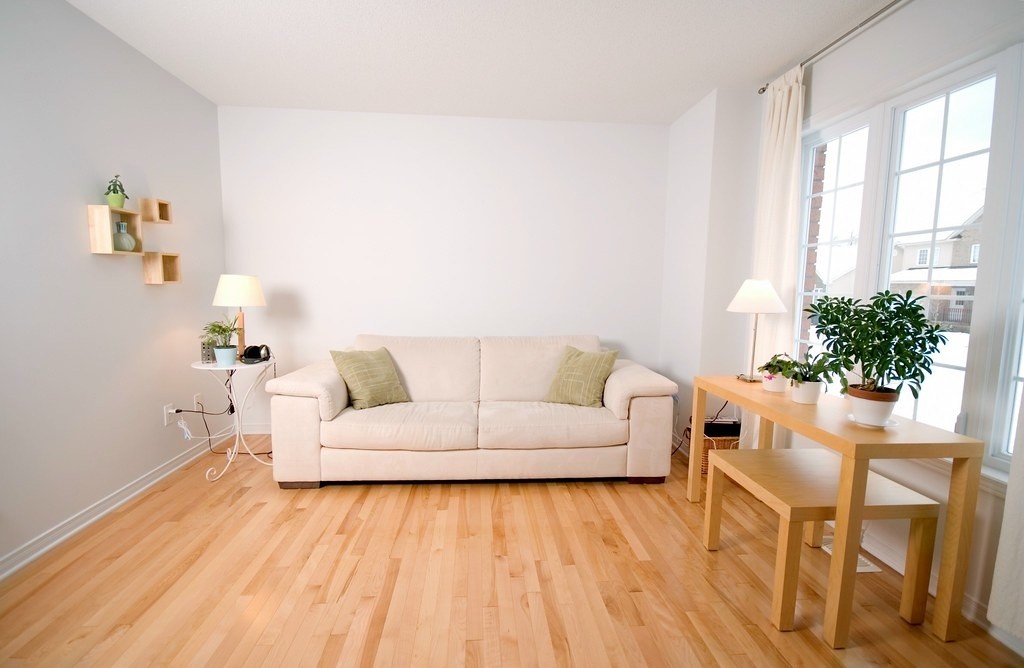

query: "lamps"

left=212, top=274, right=267, bottom=359
left=727, top=279, right=787, bottom=382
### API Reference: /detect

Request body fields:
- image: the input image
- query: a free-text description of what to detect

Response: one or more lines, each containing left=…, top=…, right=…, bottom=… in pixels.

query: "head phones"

left=240, top=345, right=270, bottom=364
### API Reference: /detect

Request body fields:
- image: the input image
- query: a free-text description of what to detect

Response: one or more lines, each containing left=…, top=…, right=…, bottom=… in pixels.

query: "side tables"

left=191, top=357, right=276, bottom=481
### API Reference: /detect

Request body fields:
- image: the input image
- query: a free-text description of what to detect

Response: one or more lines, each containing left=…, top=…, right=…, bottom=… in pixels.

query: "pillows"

left=545, top=346, right=620, bottom=408
left=330, top=347, right=409, bottom=409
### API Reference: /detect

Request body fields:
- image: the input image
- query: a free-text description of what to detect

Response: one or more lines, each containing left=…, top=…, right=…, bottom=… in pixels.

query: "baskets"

left=686, top=431, right=739, bottom=474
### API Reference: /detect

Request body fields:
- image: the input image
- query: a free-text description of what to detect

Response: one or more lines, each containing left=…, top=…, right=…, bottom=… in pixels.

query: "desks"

left=687, top=375, right=985, bottom=650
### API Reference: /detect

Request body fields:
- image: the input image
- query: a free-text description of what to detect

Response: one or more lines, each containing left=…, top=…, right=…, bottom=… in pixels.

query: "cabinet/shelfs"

left=88, top=198, right=182, bottom=285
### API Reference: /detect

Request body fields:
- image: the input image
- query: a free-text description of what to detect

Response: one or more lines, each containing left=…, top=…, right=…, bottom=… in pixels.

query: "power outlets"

left=194, top=393, right=202, bottom=412
left=164, top=402, right=176, bottom=427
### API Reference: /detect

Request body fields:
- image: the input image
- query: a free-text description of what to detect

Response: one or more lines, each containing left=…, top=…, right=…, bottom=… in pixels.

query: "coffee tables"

left=703, top=448, right=941, bottom=632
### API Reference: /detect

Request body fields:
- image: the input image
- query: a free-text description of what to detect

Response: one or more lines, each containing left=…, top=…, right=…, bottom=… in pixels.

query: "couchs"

left=264, top=334, right=679, bottom=484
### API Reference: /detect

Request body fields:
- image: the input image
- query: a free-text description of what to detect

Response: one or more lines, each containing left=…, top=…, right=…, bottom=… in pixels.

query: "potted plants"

left=104, top=175, right=129, bottom=209
left=759, top=290, right=949, bottom=424
left=203, top=313, right=243, bottom=365
